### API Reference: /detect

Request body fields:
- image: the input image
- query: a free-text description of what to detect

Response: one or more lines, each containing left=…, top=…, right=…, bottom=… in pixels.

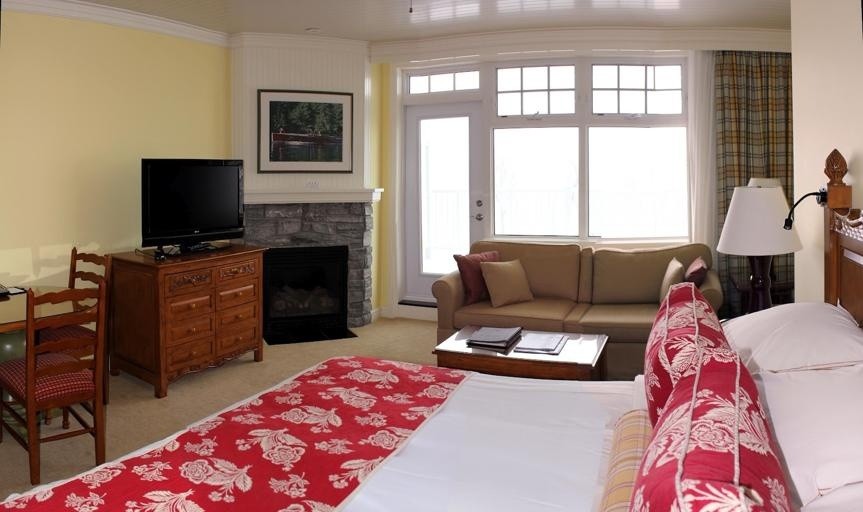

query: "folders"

left=479, top=348, right=507, bottom=353
left=513, top=333, right=570, bottom=355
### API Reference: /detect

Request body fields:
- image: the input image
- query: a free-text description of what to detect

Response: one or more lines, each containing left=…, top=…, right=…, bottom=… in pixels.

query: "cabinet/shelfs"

left=105, top=242, right=269, bottom=398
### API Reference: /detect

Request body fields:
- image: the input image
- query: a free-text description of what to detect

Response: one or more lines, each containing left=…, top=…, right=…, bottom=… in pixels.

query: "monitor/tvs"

left=141, top=157, right=246, bottom=257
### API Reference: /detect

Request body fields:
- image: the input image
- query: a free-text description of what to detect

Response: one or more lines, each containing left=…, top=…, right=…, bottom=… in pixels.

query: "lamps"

left=717, top=186, right=804, bottom=311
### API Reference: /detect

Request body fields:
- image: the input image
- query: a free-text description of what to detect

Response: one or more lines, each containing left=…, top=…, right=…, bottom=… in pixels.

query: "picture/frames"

left=256, top=89, right=354, bottom=174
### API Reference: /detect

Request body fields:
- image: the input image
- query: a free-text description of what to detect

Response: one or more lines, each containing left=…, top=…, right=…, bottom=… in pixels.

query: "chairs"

left=0, top=279, right=105, bottom=474
left=33, top=246, right=113, bottom=425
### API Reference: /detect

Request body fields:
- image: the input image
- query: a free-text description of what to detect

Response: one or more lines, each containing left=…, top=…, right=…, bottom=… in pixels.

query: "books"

left=513, top=325, right=569, bottom=355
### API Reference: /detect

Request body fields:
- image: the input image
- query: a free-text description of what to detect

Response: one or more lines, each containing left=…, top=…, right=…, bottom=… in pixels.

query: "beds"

left=1, top=148, right=863, bottom=512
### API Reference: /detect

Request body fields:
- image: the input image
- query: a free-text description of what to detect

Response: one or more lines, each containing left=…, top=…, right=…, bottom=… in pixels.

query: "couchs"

left=431, top=239, right=723, bottom=382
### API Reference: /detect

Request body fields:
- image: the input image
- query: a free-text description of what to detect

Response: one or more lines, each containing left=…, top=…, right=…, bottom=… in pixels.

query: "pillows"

left=643, top=281, right=734, bottom=429
left=628, top=347, right=792, bottom=512
left=452, top=250, right=501, bottom=305
left=755, top=360, right=863, bottom=505
left=685, top=256, right=708, bottom=289
left=659, top=256, right=684, bottom=306
left=601, top=408, right=654, bottom=512
left=720, top=301, right=863, bottom=374
left=480, top=258, right=534, bottom=308
